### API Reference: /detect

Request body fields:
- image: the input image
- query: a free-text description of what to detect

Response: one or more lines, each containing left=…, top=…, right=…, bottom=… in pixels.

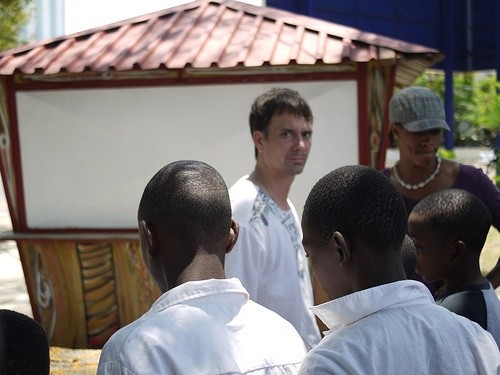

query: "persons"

left=225, top=86, right=332, bottom=353
left=97, top=160, right=308, bottom=375
left=298, top=165, right=500, bottom=375
left=401, top=234, right=436, bottom=302
left=0, top=309, right=50, bottom=375
left=383, top=86, right=500, bottom=290
left=408, top=189, right=500, bottom=350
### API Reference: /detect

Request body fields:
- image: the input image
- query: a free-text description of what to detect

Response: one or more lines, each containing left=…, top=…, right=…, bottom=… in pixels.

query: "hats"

left=389, top=86, right=451, bottom=132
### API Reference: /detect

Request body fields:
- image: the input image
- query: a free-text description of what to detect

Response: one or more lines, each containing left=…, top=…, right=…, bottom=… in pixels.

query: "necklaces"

left=393, top=156, right=442, bottom=189
